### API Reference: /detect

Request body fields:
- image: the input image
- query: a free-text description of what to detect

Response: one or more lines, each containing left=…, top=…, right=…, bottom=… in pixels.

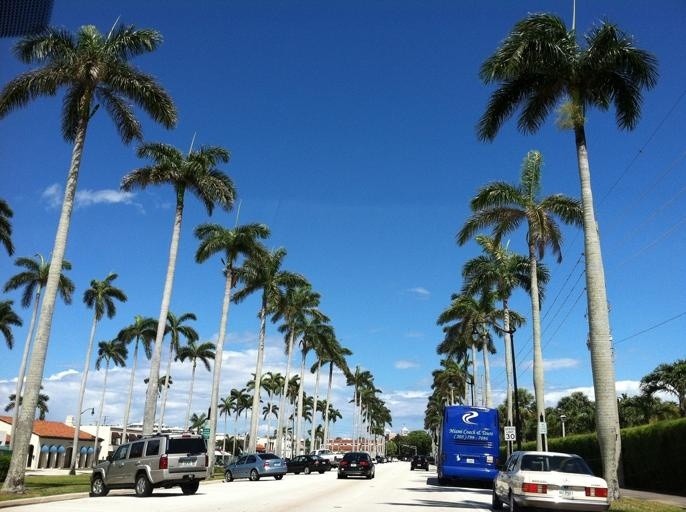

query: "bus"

left=436, top=404, right=500, bottom=488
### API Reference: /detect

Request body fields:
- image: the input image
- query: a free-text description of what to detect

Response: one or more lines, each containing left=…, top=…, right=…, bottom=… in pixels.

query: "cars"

left=410, top=455, right=429, bottom=472
left=371, top=454, right=399, bottom=464
left=285, top=454, right=332, bottom=475
left=336, top=452, right=375, bottom=480
left=310, top=448, right=344, bottom=469
left=490, top=450, right=611, bottom=512
left=223, top=453, right=289, bottom=482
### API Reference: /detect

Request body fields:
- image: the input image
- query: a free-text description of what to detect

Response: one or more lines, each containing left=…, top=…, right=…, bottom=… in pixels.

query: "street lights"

left=560, top=412, right=568, bottom=437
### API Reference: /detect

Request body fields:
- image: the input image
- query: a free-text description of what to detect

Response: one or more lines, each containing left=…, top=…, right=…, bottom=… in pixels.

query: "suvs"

left=88, top=431, right=209, bottom=497
